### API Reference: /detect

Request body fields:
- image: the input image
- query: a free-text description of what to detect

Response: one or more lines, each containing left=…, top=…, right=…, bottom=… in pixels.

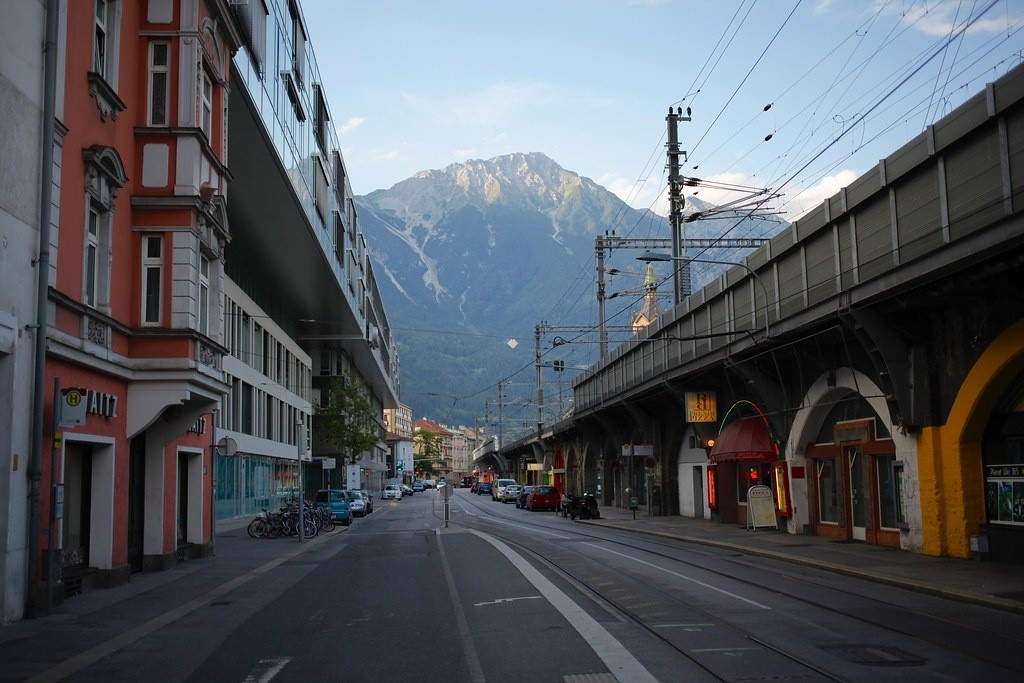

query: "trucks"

left=460, top=476, right=475, bottom=487
left=421, top=480, right=435, bottom=486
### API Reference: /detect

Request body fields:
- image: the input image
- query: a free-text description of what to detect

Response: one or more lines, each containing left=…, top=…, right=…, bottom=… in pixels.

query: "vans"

left=492, top=479, right=517, bottom=501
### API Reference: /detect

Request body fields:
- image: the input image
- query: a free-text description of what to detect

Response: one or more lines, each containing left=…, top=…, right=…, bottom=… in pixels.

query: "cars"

left=399, top=485, right=413, bottom=497
left=346, top=490, right=367, bottom=517
left=382, top=485, right=402, bottom=500
left=526, top=486, right=561, bottom=512
left=413, top=483, right=424, bottom=492
left=423, top=483, right=432, bottom=488
left=477, top=483, right=492, bottom=495
left=471, top=482, right=482, bottom=494
left=437, top=482, right=446, bottom=490
left=314, top=489, right=353, bottom=525
left=516, top=486, right=533, bottom=508
left=361, top=491, right=373, bottom=513
left=502, top=485, right=523, bottom=504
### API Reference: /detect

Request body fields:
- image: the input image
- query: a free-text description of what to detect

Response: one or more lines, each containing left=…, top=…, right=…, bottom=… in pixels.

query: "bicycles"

left=247, top=500, right=335, bottom=538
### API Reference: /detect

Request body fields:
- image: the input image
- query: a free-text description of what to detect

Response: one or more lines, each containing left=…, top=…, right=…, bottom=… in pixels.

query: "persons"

left=561, top=492, right=567, bottom=500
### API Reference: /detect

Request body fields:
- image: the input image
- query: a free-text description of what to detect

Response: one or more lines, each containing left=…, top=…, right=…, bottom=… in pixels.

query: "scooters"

left=561, top=491, right=600, bottom=520
